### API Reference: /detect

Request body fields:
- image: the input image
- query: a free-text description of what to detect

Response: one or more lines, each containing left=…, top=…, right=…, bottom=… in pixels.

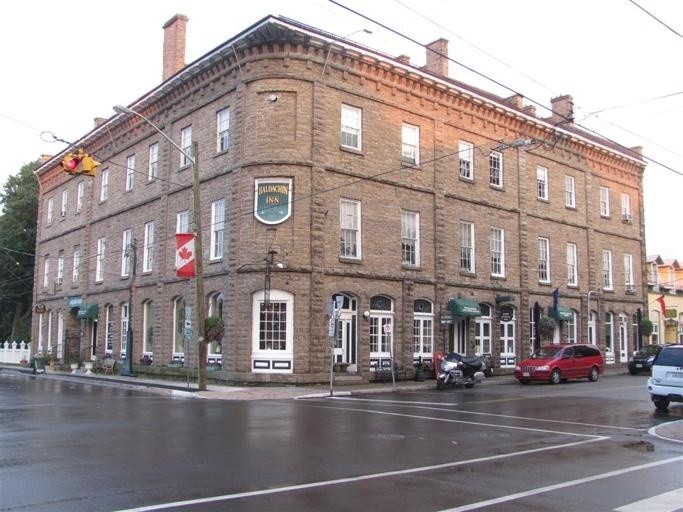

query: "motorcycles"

left=435, top=353, right=486, bottom=390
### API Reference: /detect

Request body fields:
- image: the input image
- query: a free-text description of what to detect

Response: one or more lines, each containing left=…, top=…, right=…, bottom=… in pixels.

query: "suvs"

left=647, top=345, right=683, bottom=411
left=628, top=344, right=664, bottom=374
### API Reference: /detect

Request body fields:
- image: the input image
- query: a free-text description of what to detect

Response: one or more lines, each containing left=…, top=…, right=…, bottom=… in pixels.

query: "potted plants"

left=82, top=359, right=95, bottom=375
left=69, top=359, right=79, bottom=374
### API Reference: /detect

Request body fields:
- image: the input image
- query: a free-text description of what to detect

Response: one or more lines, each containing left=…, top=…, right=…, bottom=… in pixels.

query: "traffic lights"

left=83, top=157, right=100, bottom=176
left=60, top=153, right=82, bottom=176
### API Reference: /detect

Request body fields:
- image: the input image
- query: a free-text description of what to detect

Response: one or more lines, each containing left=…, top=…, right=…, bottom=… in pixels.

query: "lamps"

left=362, top=310, right=369, bottom=320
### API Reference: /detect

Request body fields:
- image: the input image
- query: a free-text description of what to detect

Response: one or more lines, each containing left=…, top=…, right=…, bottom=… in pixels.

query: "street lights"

left=111, top=105, right=208, bottom=391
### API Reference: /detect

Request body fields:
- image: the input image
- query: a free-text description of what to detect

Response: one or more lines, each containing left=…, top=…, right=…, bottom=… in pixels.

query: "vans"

left=513, top=343, right=605, bottom=385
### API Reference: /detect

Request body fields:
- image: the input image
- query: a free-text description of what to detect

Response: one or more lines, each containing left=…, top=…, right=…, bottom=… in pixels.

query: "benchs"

left=373, top=361, right=398, bottom=384
left=94, top=357, right=115, bottom=375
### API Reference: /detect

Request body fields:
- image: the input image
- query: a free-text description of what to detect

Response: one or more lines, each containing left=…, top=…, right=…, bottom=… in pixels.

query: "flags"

left=655, top=295, right=666, bottom=317
left=553, top=288, right=559, bottom=312
left=174, top=233, right=196, bottom=277
